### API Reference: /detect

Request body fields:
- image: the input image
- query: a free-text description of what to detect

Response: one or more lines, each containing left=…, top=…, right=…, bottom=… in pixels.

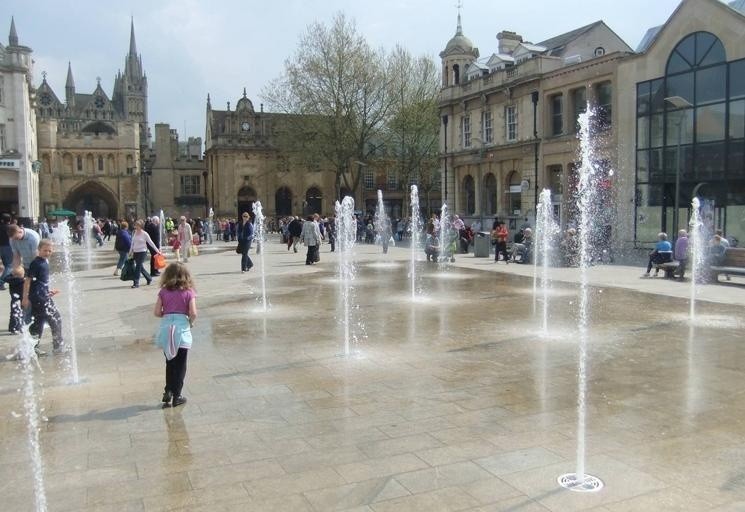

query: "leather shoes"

left=152, top=272, right=160, bottom=276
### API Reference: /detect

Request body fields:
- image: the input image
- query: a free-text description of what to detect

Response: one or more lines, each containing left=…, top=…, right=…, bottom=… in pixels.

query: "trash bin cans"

left=474, top=231, right=490, bottom=257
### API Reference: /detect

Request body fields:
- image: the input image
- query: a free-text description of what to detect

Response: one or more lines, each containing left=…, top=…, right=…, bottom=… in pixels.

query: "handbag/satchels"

left=191, top=245, right=197, bottom=256
left=120, top=257, right=136, bottom=281
left=236, top=242, right=242, bottom=253
left=154, top=253, right=166, bottom=270
left=492, top=239, right=497, bottom=245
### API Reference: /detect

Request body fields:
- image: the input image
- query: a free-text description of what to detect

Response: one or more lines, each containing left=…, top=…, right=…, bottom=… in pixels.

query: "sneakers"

left=173, top=395, right=187, bottom=406
left=114, top=270, right=121, bottom=276
left=11, top=330, right=20, bottom=335
left=644, top=272, right=650, bottom=276
left=34, top=346, right=47, bottom=356
left=653, top=272, right=658, bottom=276
left=162, top=390, right=172, bottom=403
left=147, top=280, right=152, bottom=286
left=132, top=284, right=139, bottom=288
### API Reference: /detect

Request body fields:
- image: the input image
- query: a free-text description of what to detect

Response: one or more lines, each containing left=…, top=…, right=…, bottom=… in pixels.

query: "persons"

left=669, top=229, right=689, bottom=280
left=643, top=232, right=672, bottom=277
left=708, top=228, right=730, bottom=280
left=153, top=260, right=197, bottom=407
left=705, top=234, right=727, bottom=265
left=396, top=213, right=615, bottom=267
left=0, top=208, right=72, bottom=357
left=75, top=211, right=374, bottom=288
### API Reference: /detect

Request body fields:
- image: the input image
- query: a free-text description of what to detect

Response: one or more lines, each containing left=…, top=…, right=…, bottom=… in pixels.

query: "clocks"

left=242, top=122, right=250, bottom=131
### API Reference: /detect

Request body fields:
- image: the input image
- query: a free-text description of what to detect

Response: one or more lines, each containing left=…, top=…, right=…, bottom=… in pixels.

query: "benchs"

left=651, top=248, right=745, bottom=282
left=505, top=233, right=523, bottom=255
